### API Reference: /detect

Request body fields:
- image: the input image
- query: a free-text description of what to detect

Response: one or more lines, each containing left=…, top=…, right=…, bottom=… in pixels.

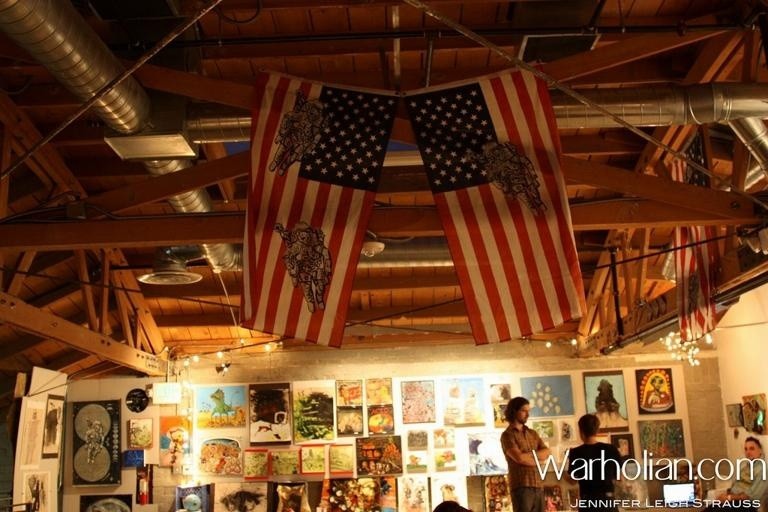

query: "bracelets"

left=727, top=495, right=732, bottom=502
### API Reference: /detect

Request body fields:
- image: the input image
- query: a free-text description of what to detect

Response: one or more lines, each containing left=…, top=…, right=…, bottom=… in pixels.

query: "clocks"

left=125, top=389, right=148, bottom=413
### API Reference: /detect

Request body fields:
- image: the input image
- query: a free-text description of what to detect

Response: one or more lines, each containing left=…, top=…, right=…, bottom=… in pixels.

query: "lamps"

left=146, top=382, right=180, bottom=404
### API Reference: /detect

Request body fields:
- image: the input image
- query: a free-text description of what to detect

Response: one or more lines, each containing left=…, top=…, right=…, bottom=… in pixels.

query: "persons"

left=643, top=375, right=670, bottom=408
left=26, top=475, right=36, bottom=504
left=545, top=487, right=563, bottom=512
left=701, top=437, right=768, bottom=512
left=566, top=414, right=623, bottom=512
left=500, top=396, right=550, bottom=512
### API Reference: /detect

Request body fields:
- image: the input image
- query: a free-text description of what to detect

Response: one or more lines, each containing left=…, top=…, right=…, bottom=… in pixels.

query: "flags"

left=671, top=124, right=717, bottom=343
left=401, top=59, right=589, bottom=346
left=238, top=69, right=397, bottom=351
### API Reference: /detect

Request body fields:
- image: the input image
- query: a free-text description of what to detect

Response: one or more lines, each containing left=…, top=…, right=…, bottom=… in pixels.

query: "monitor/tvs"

left=662, top=482, right=696, bottom=507
